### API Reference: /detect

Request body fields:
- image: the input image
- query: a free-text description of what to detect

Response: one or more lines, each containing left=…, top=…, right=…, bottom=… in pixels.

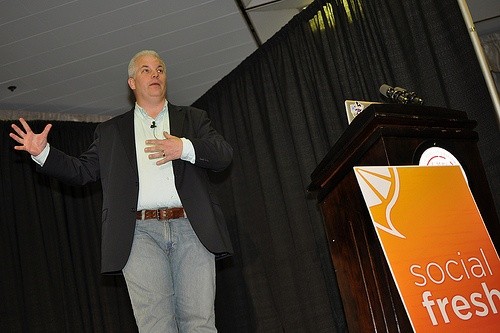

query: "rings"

left=162, top=151, right=166, bottom=156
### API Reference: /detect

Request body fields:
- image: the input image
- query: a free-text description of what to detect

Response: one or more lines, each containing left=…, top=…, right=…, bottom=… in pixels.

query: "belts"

left=136, top=207, right=184, bottom=221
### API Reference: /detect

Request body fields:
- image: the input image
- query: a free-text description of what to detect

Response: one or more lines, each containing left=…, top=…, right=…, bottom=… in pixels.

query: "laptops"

left=344, top=100, right=386, bottom=126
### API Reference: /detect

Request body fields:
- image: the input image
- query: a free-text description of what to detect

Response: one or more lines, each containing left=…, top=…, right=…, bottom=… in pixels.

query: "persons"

left=10, top=50, right=235, bottom=333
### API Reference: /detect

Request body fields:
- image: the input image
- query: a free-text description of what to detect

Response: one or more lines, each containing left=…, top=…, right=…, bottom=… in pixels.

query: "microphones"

left=379, top=84, right=408, bottom=102
left=394, top=87, right=423, bottom=104
left=150, top=121, right=157, bottom=128
